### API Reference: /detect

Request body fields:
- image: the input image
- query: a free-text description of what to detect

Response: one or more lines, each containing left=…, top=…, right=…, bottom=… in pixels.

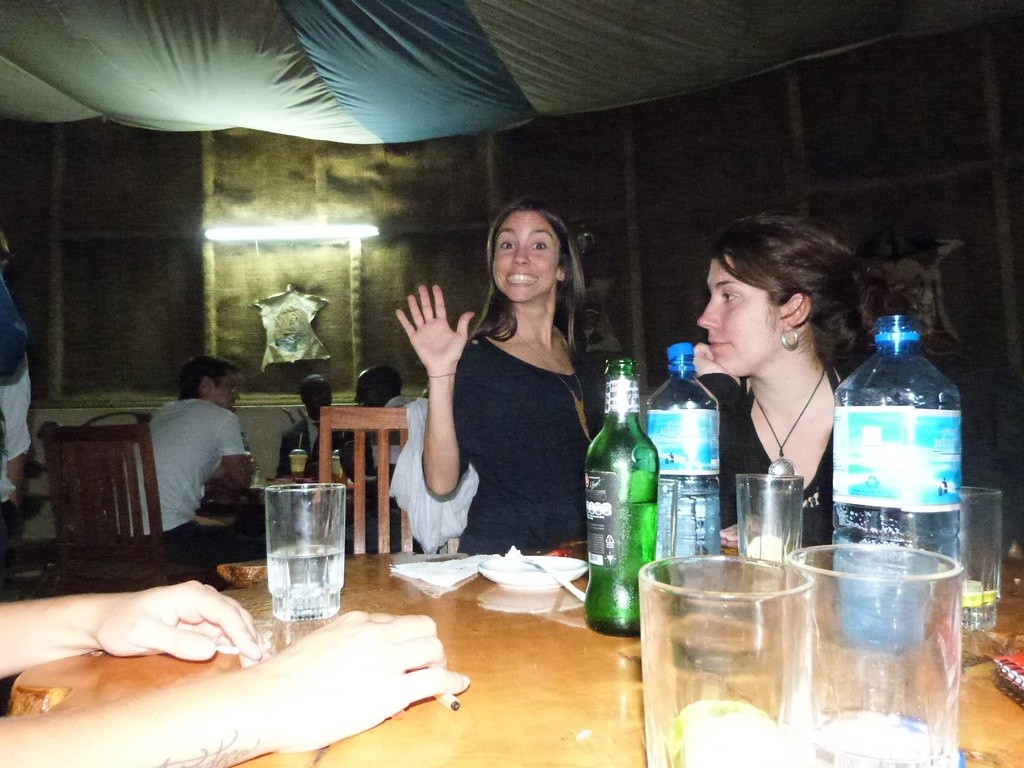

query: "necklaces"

left=756, top=369, right=826, bottom=458
left=517, top=332, right=592, bottom=442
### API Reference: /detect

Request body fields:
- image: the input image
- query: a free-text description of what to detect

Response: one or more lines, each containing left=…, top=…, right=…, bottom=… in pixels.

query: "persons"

left=115, top=357, right=265, bottom=568
left=691, top=214, right=989, bottom=549
left=0, top=234, right=32, bottom=520
left=395, top=198, right=605, bottom=557
left=0, top=580, right=470, bottom=768
left=340, top=367, right=414, bottom=549
left=276, top=374, right=374, bottom=478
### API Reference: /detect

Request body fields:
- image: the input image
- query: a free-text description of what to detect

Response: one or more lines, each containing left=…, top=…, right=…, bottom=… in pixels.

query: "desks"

left=6, top=551, right=1024, bottom=768
left=197, top=477, right=352, bottom=517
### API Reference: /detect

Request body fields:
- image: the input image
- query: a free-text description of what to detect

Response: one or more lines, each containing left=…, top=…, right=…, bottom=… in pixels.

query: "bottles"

left=582, top=355, right=661, bottom=638
left=832, top=314, right=963, bottom=656
left=645, top=342, right=723, bottom=562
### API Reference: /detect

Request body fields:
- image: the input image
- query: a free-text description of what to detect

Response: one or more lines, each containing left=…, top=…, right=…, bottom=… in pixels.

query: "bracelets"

left=428, top=373, right=456, bottom=378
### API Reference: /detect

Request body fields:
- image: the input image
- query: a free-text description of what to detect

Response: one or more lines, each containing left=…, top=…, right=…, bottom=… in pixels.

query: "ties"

left=312, top=422, right=320, bottom=458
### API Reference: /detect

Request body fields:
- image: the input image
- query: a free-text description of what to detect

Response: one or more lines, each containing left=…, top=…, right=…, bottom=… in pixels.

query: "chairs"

left=316, top=404, right=460, bottom=555
left=39, top=419, right=229, bottom=598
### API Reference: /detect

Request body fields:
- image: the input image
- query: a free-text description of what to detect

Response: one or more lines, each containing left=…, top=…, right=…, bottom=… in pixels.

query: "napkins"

left=387, top=553, right=502, bottom=589
left=389, top=572, right=482, bottom=599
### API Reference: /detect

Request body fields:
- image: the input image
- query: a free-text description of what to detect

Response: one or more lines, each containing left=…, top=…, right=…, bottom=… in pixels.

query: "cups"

left=264, top=483, right=347, bottom=623
left=288, top=449, right=309, bottom=481
left=635, top=554, right=817, bottom=768
left=958, top=486, right=1003, bottom=634
left=784, top=543, right=963, bottom=768
left=735, top=473, right=805, bottom=561
left=330, top=449, right=349, bottom=485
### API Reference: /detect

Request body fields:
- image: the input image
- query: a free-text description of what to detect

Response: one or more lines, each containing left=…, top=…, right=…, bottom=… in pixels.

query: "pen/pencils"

left=434, top=693, right=460, bottom=711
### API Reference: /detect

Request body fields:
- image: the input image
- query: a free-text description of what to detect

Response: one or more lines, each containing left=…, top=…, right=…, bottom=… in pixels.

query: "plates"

left=477, top=555, right=589, bottom=590
